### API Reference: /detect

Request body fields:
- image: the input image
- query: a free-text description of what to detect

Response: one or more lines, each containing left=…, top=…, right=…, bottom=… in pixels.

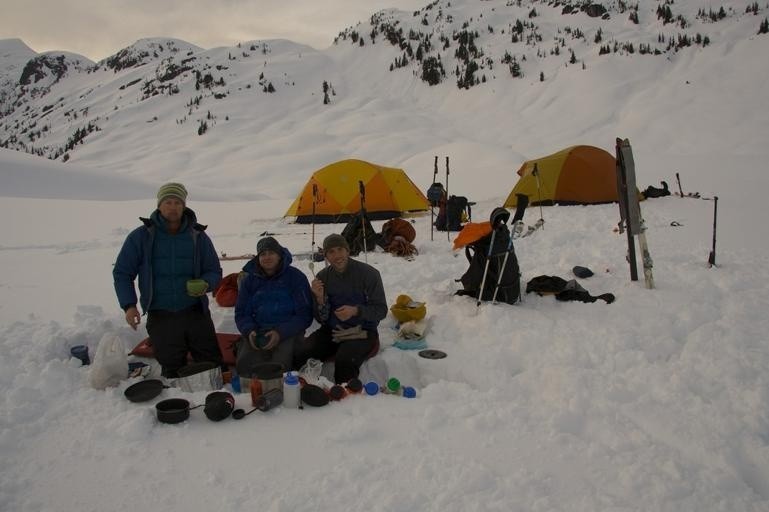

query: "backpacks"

left=435, top=196, right=467, bottom=231
left=341, top=208, right=377, bottom=256
left=460, top=225, right=519, bottom=304
left=427, top=183, right=447, bottom=207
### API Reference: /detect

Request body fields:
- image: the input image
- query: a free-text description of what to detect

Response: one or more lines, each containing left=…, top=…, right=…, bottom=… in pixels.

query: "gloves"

left=332, top=324, right=369, bottom=343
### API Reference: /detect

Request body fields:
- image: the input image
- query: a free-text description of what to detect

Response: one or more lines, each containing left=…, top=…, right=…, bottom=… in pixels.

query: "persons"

left=113, top=181, right=225, bottom=374
left=234, top=236, right=316, bottom=377
left=297, top=231, right=393, bottom=384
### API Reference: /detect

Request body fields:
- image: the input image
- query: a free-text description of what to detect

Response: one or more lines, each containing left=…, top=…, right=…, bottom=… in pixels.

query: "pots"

left=154, top=396, right=208, bottom=423
left=123, top=378, right=164, bottom=402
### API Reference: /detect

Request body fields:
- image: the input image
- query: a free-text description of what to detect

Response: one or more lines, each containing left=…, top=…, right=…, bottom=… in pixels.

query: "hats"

left=323, top=234, right=350, bottom=257
left=491, top=208, right=510, bottom=229
left=157, top=182, right=187, bottom=209
left=257, top=237, right=283, bottom=259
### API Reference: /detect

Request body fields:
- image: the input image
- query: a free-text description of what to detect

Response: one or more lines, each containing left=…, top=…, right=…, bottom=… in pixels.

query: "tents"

left=279, top=156, right=436, bottom=222
left=497, top=143, right=640, bottom=208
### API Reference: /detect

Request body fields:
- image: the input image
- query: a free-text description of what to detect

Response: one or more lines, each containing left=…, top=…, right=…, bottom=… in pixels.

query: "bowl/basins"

left=252, top=335, right=270, bottom=348
left=186, top=279, right=208, bottom=297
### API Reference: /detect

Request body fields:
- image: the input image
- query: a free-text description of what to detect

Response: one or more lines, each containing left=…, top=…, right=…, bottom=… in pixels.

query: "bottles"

left=230, top=363, right=418, bottom=412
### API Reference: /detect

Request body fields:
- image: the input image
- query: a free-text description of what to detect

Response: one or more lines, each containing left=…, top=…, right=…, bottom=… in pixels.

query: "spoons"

left=308, top=261, right=317, bottom=280
